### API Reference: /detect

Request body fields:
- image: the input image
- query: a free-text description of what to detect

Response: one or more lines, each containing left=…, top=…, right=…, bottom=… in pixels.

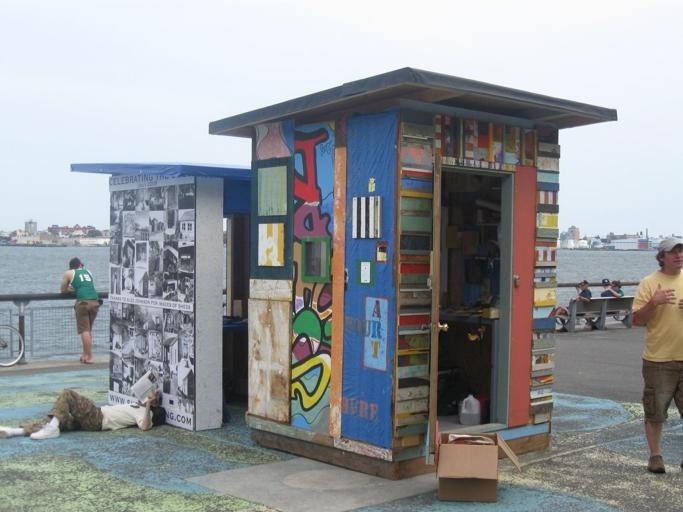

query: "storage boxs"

left=432, top=421, right=521, bottom=502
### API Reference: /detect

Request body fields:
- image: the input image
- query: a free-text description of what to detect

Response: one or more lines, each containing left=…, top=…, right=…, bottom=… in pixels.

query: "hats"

left=68, top=257, right=83, bottom=269
left=577, top=279, right=588, bottom=286
left=656, top=236, right=683, bottom=253
left=601, top=278, right=609, bottom=285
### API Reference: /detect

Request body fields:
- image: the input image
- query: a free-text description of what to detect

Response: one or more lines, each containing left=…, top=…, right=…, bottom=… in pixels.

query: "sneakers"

left=0, top=425, right=13, bottom=438
left=647, top=455, right=665, bottom=473
left=30, top=423, right=60, bottom=440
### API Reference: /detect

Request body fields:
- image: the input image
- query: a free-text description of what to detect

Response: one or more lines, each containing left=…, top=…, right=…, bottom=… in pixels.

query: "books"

left=130, top=369, right=161, bottom=403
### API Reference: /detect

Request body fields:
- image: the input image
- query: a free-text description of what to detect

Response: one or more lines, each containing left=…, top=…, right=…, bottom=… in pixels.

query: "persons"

left=557, top=279, right=624, bottom=332
left=59, top=256, right=100, bottom=364
left=630, top=237, right=683, bottom=473
left=0, top=389, right=165, bottom=440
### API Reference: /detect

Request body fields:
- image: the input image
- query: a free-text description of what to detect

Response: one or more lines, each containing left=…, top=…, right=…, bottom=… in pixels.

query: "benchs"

left=559, top=295, right=636, bottom=335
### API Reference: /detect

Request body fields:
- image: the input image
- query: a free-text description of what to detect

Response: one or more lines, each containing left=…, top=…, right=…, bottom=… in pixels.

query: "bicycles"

left=0, top=326, right=24, bottom=367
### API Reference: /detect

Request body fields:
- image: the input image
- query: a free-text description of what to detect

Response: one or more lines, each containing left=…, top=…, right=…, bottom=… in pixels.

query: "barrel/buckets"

left=458, top=395, right=480, bottom=426
